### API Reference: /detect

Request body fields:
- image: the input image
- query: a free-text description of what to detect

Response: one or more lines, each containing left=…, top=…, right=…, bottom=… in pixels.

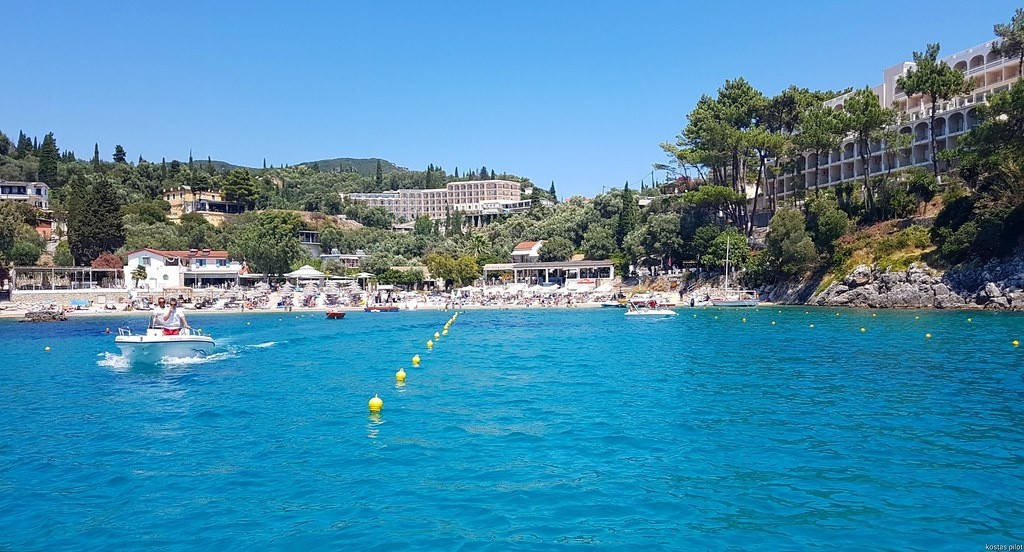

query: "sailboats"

left=707, top=233, right=759, bottom=307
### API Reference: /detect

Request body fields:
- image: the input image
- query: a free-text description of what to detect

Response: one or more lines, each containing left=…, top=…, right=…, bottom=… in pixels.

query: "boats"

left=601, top=292, right=678, bottom=317
left=114, top=326, right=215, bottom=364
left=363, top=305, right=399, bottom=313
left=325, top=312, right=346, bottom=319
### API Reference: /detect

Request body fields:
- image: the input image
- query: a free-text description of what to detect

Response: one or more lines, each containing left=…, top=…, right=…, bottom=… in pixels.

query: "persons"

left=37, top=291, right=416, bottom=311
left=102, top=327, right=114, bottom=334
left=487, top=267, right=610, bottom=284
left=152, top=297, right=191, bottom=335
left=653, top=266, right=657, bottom=275
left=436, top=289, right=623, bottom=309
left=218, top=278, right=283, bottom=291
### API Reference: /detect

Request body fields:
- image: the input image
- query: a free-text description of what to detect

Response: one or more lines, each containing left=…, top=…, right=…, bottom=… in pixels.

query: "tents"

left=163, top=286, right=193, bottom=302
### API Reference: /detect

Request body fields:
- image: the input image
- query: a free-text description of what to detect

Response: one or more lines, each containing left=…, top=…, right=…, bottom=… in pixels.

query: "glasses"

left=171, top=304, right=177, bottom=306
left=159, top=300, right=165, bottom=304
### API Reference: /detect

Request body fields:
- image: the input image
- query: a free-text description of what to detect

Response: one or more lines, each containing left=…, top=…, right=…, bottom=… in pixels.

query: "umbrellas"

left=194, top=282, right=420, bottom=300
left=613, top=282, right=627, bottom=293
